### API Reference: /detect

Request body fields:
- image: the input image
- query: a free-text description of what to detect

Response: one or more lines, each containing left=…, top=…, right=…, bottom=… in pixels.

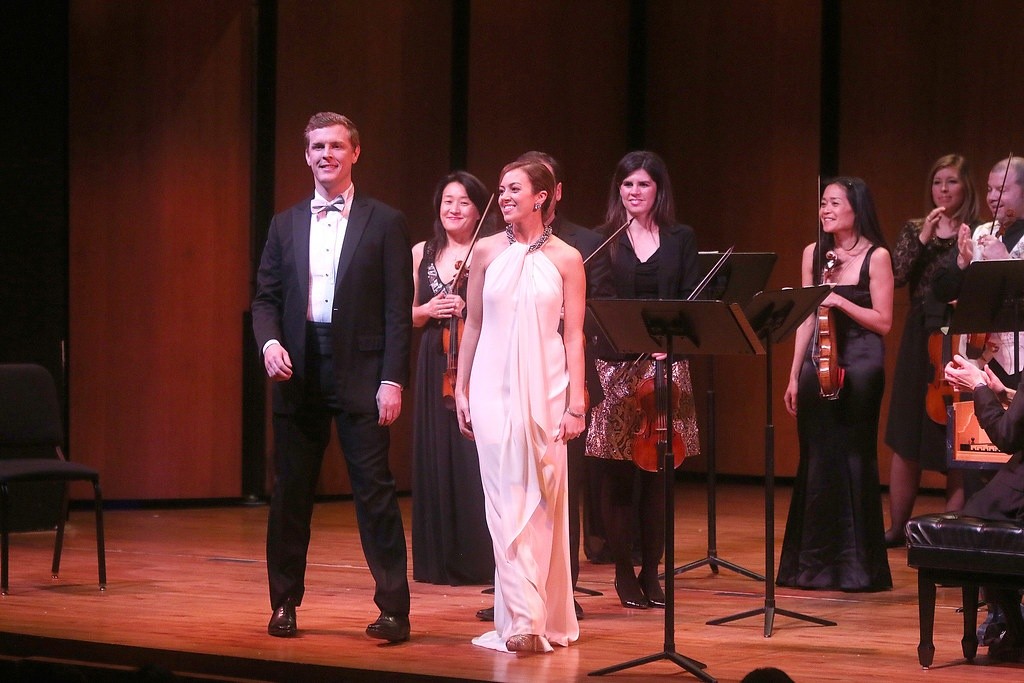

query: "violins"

left=563, top=331, right=591, bottom=415
left=629, top=358, right=688, bottom=473
left=440, top=259, right=468, bottom=403
left=927, top=206, right=1016, bottom=428
left=817, top=249, right=840, bottom=401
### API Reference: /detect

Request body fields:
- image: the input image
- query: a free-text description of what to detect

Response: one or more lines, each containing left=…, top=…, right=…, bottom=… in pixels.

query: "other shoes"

left=506, top=634, right=537, bottom=652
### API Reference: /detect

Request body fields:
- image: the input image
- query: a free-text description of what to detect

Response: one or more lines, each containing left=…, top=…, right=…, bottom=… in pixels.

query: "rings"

left=439, top=310, right=442, bottom=314
left=577, top=434, right=581, bottom=438
left=926, top=216, right=931, bottom=222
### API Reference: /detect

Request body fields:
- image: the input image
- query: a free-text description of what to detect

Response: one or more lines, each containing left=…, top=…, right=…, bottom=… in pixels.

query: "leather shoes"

left=366, top=610, right=410, bottom=641
left=268, top=605, right=297, bottom=636
left=476, top=607, right=494, bottom=619
left=574, top=601, right=583, bottom=618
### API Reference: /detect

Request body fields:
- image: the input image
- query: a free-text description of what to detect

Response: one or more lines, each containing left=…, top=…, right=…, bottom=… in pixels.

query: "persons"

left=518, top=150, right=700, bottom=606
left=778, top=177, right=896, bottom=594
left=454, top=160, right=585, bottom=655
left=411, top=169, right=496, bottom=588
left=250, top=111, right=415, bottom=644
left=944, top=353, right=1024, bottom=660
left=947, top=155, right=1024, bottom=484
left=884, top=152, right=984, bottom=549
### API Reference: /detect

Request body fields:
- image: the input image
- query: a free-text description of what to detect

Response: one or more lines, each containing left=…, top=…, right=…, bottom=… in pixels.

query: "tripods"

left=578, top=251, right=838, bottom=683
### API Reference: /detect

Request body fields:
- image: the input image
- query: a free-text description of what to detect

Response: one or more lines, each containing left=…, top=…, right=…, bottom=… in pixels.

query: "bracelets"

left=566, top=408, right=586, bottom=418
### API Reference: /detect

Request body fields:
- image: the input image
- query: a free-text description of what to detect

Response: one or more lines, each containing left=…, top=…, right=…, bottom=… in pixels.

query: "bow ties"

left=311, top=194, right=345, bottom=214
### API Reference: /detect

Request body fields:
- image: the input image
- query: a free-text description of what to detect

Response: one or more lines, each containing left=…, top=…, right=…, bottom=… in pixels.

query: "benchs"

left=906, top=513, right=1024, bottom=668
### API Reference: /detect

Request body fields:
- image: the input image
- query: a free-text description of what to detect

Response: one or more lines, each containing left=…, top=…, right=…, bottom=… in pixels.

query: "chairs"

left=0, top=364, right=109, bottom=595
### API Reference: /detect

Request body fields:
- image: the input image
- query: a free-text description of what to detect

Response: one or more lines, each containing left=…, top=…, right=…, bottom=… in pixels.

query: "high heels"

left=638, top=572, right=666, bottom=607
left=614, top=577, right=647, bottom=608
